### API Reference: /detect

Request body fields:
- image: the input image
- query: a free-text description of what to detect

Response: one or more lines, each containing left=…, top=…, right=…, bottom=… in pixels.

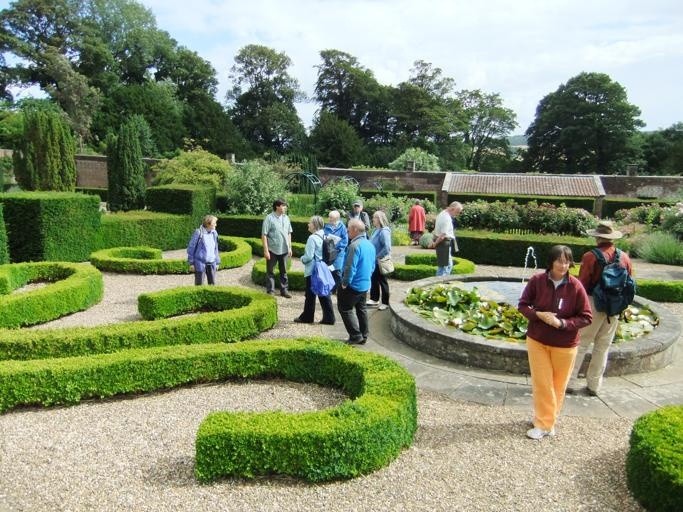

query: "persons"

left=409, top=199, right=427, bottom=245
left=186, top=215, right=220, bottom=285
left=293, top=199, right=392, bottom=345
left=261, top=199, right=294, bottom=299
left=564, top=221, right=632, bottom=396
left=428, top=201, right=463, bottom=276
left=517, top=244, right=593, bottom=440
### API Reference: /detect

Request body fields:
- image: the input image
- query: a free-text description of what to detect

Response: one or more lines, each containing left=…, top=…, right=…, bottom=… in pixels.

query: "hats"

left=586, top=219, right=623, bottom=241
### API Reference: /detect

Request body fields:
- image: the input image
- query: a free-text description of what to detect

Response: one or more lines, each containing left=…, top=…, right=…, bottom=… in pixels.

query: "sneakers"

left=585, top=387, right=597, bottom=397
left=293, top=318, right=303, bottom=323
left=319, top=319, right=335, bottom=325
left=525, top=426, right=555, bottom=439
left=411, top=241, right=420, bottom=246
left=377, top=304, right=388, bottom=311
left=280, top=291, right=293, bottom=299
left=345, top=336, right=368, bottom=346
left=366, top=299, right=381, bottom=306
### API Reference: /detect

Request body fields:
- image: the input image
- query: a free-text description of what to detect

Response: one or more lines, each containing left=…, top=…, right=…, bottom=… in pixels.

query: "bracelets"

left=561, top=319, right=566, bottom=329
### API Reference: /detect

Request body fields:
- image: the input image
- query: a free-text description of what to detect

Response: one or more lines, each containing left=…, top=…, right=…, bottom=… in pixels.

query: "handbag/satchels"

left=376, top=254, right=396, bottom=276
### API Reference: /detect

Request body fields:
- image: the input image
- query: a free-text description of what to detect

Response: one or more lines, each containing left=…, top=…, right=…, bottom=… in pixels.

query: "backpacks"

left=313, top=232, right=338, bottom=266
left=589, top=246, right=635, bottom=325
